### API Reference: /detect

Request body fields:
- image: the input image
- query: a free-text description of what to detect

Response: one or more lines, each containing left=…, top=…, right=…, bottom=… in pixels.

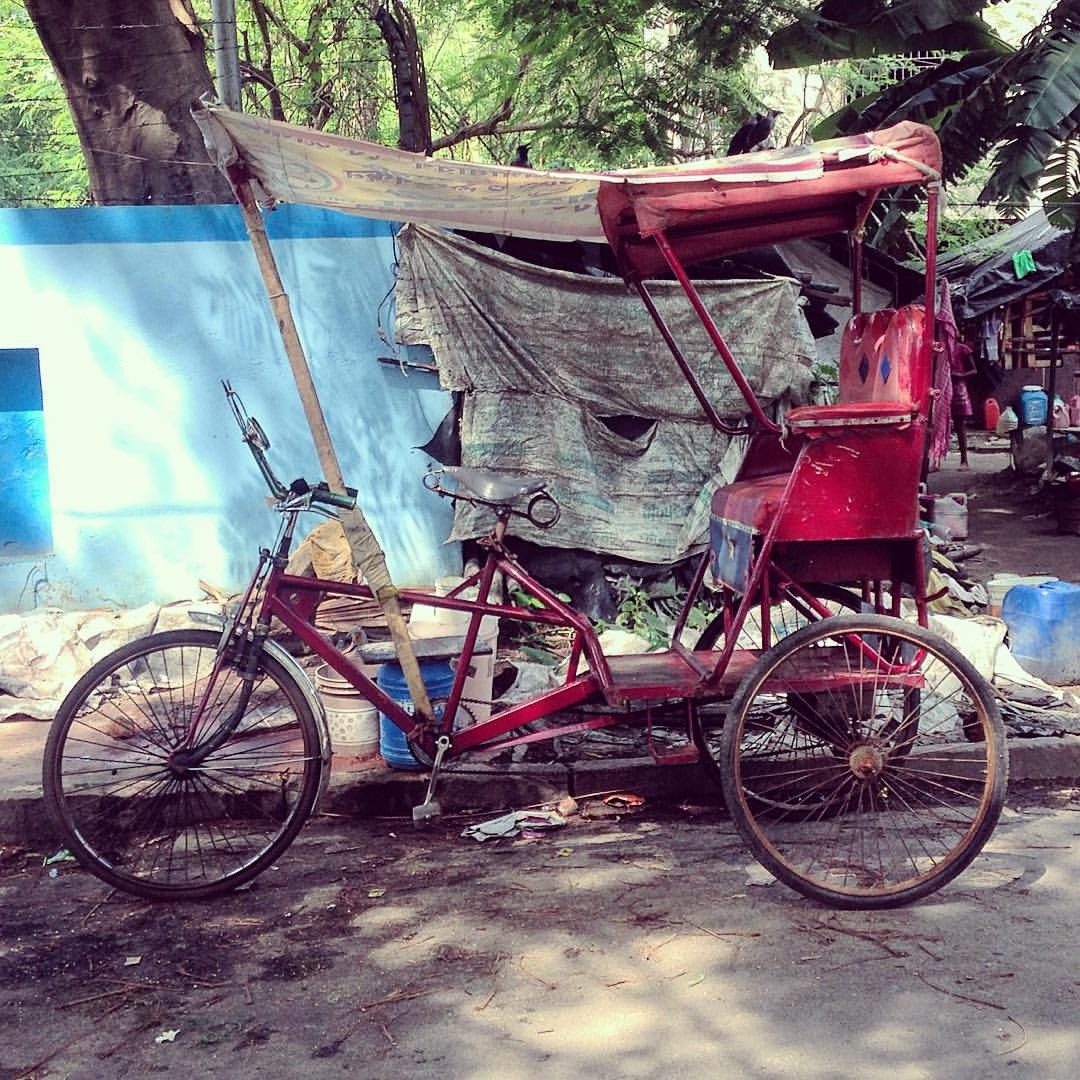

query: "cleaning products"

left=1068, top=392, right=1080, bottom=426
left=1053, top=394, right=1069, bottom=427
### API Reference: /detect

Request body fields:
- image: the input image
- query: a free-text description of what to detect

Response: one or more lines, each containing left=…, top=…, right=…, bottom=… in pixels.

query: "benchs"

left=703, top=303, right=929, bottom=661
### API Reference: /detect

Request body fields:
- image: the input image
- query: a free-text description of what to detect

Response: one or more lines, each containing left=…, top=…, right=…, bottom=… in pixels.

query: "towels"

left=976, top=311, right=997, bottom=339
left=976, top=320, right=1001, bottom=362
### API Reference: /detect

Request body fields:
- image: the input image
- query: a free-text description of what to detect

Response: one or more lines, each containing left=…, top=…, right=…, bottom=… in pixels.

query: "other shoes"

left=928, top=463, right=940, bottom=472
left=959, top=460, right=968, bottom=471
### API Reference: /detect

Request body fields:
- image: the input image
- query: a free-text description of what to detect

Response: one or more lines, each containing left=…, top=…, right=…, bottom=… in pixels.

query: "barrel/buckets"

left=1016, top=385, right=1048, bottom=425
left=931, top=492, right=969, bottom=541
left=988, top=576, right=1080, bottom=688
left=376, top=658, right=456, bottom=772
left=314, top=661, right=380, bottom=759
left=982, top=394, right=1000, bottom=430
left=406, top=620, right=500, bottom=732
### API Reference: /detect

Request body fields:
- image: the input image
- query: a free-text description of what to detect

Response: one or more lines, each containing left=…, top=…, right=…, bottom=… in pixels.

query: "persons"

left=949, top=341, right=978, bottom=473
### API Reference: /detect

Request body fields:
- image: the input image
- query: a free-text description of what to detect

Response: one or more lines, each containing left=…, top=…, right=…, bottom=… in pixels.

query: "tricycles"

left=40, top=116, right=1010, bottom=910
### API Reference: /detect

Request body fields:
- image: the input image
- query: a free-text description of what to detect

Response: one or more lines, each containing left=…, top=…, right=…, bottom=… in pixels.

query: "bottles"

left=1050, top=393, right=1070, bottom=428
left=996, top=406, right=1018, bottom=438
left=1069, top=392, right=1080, bottom=427
